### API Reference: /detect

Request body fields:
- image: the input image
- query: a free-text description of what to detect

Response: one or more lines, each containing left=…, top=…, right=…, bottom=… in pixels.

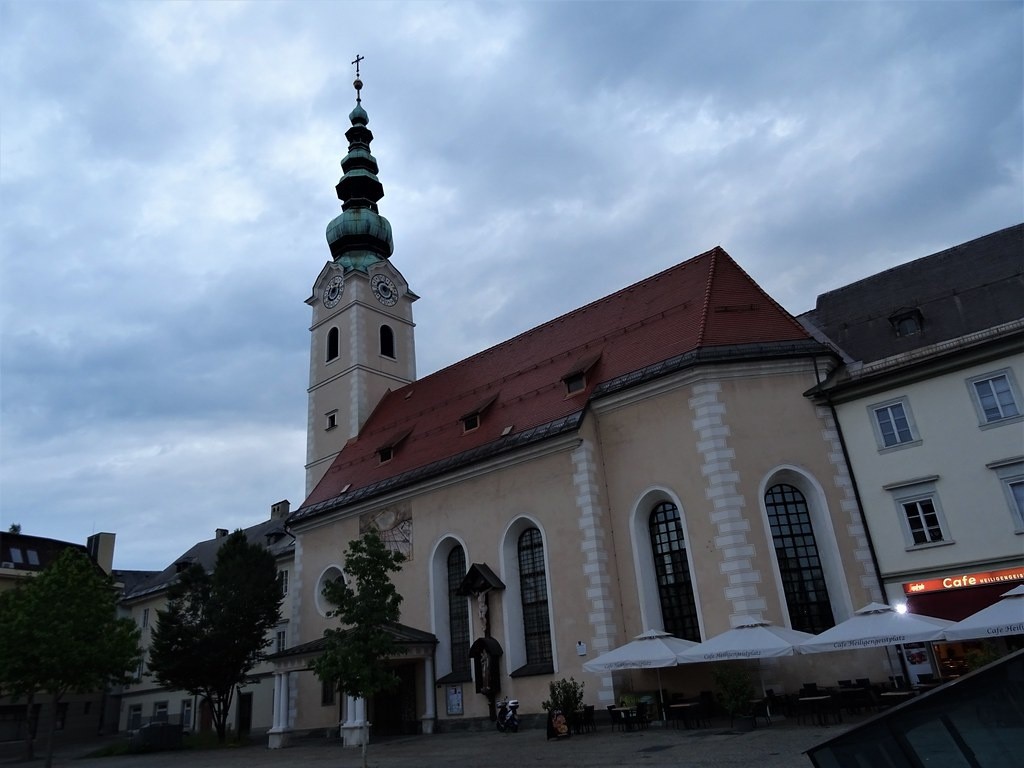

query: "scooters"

left=495, top=696, right=520, bottom=732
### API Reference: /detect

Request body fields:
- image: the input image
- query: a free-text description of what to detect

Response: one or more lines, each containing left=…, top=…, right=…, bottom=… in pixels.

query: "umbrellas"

left=582, top=582, right=1024, bottom=727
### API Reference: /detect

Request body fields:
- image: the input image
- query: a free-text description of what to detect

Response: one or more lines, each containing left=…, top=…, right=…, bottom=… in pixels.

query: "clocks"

left=322, top=275, right=345, bottom=309
left=370, top=274, right=398, bottom=307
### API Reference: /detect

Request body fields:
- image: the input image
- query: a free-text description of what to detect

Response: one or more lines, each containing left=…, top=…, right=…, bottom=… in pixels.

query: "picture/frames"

left=445, top=684, right=464, bottom=715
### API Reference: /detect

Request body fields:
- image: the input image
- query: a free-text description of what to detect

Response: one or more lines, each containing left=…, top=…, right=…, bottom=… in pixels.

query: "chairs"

left=545, top=673, right=966, bottom=740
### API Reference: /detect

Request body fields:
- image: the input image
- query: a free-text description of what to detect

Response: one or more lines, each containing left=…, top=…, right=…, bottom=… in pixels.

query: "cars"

left=128, top=722, right=194, bottom=738
